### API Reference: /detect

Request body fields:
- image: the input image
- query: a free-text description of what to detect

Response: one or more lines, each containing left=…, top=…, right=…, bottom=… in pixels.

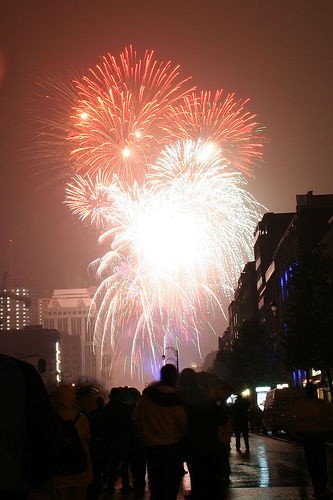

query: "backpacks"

left=48, top=412, right=82, bottom=473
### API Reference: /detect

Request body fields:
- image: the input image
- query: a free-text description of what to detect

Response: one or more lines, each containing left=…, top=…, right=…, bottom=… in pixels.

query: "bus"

left=262, top=388, right=321, bottom=436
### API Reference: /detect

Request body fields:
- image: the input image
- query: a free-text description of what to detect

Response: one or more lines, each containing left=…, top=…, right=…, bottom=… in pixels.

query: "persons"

left=1, top=351, right=333, bottom=500
